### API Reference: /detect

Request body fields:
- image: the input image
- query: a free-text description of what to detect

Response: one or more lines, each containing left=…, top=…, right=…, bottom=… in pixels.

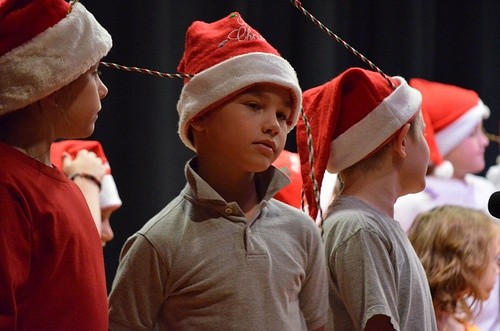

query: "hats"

left=50, top=140, right=122, bottom=211
left=0, top=0, right=113, bottom=116
left=410, top=79, right=489, bottom=179
left=177, top=11, right=302, bottom=151
left=274, top=151, right=308, bottom=212
left=295, top=67, right=424, bottom=222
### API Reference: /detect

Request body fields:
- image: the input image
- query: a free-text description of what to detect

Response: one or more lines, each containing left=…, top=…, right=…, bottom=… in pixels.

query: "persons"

left=108, top=12, right=328, bottom=331
left=0, top=0, right=107, bottom=331
left=50, top=140, right=122, bottom=246
left=271, top=150, right=342, bottom=226
left=394, top=78, right=500, bottom=331
left=297, top=68, right=438, bottom=331
left=407, top=204, right=500, bottom=331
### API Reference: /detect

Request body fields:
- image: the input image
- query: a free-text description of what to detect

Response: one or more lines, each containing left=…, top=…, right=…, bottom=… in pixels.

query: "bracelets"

left=71, top=173, right=102, bottom=191
